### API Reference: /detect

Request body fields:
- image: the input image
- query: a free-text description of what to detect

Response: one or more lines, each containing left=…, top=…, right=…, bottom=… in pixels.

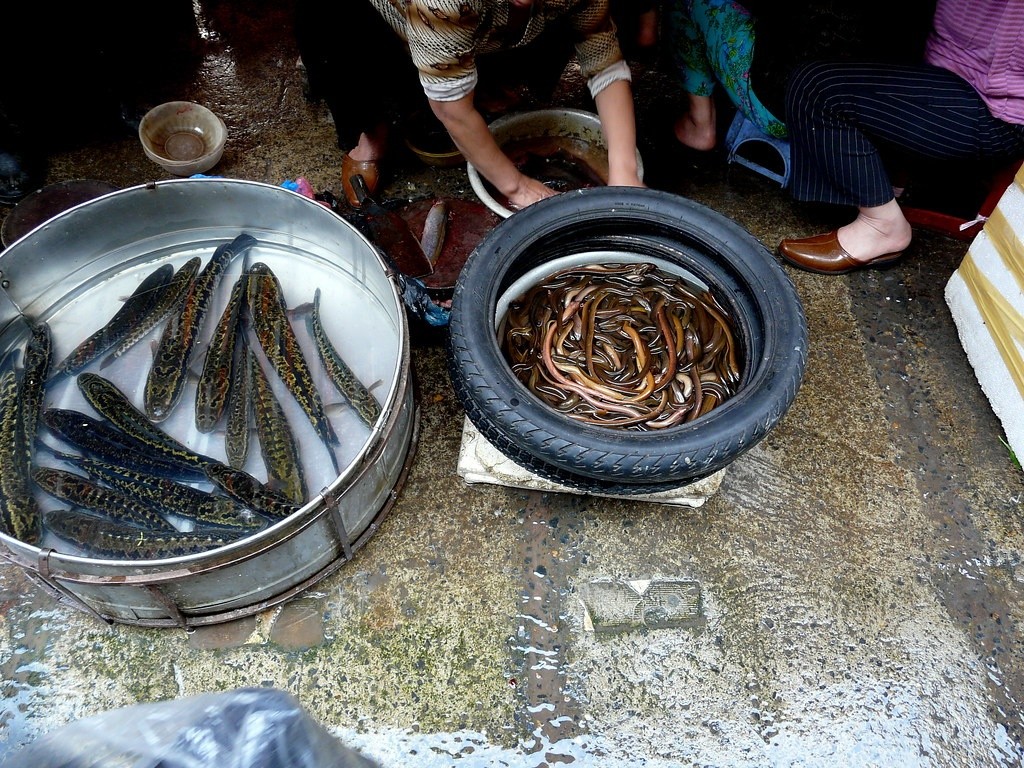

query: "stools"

left=725, top=109, right=795, bottom=189
left=900, top=156, right=1024, bottom=237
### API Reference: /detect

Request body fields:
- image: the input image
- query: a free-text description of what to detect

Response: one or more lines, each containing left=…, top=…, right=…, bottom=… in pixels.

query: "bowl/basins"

left=138, top=101, right=227, bottom=176
left=404, top=110, right=465, bottom=167
left=0, top=177, right=416, bottom=617
left=466, top=106, right=643, bottom=219
left=493, top=251, right=710, bottom=337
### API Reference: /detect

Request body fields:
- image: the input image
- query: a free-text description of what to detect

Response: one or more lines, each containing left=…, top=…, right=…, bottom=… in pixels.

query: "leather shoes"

left=342, top=153, right=379, bottom=209
left=779, top=225, right=902, bottom=272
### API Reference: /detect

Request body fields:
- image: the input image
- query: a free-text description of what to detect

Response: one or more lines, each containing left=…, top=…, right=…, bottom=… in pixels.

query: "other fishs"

left=419, top=200, right=448, bottom=266
left=507, top=264, right=742, bottom=432
left=0, top=232, right=384, bottom=561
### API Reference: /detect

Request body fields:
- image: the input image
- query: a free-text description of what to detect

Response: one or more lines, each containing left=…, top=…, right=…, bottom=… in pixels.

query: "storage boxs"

left=944, top=161, right=1024, bottom=470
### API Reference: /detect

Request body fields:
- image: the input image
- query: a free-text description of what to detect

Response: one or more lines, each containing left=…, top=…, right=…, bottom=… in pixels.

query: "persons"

left=301, top=0, right=648, bottom=210
left=0, top=0, right=201, bottom=212
left=607, top=0, right=1024, bottom=275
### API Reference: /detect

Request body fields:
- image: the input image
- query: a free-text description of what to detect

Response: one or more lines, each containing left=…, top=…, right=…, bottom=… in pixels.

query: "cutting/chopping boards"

left=395, top=196, right=501, bottom=290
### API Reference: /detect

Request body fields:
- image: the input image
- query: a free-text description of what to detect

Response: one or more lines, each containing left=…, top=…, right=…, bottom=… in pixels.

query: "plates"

left=0, top=179, right=122, bottom=249
